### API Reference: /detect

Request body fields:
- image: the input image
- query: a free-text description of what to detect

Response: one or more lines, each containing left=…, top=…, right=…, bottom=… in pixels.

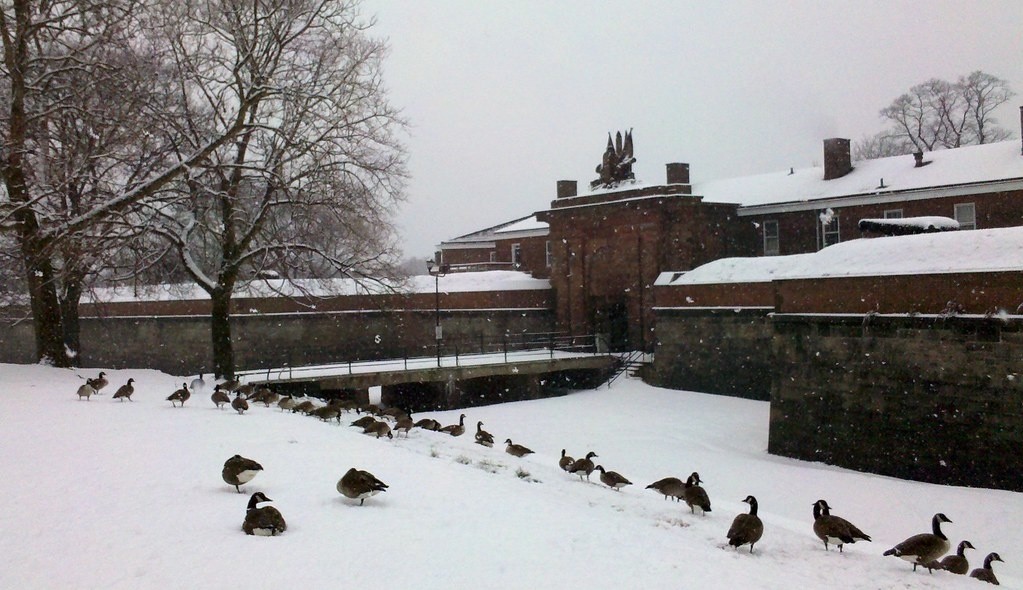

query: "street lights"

left=425, top=250, right=450, bottom=369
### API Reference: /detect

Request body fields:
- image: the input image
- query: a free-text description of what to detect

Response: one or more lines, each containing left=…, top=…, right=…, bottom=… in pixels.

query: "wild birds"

left=811, top=499, right=872, bottom=555
left=76, top=371, right=135, bottom=404
left=165, top=372, right=764, bottom=556
left=882, top=513, right=1004, bottom=586
